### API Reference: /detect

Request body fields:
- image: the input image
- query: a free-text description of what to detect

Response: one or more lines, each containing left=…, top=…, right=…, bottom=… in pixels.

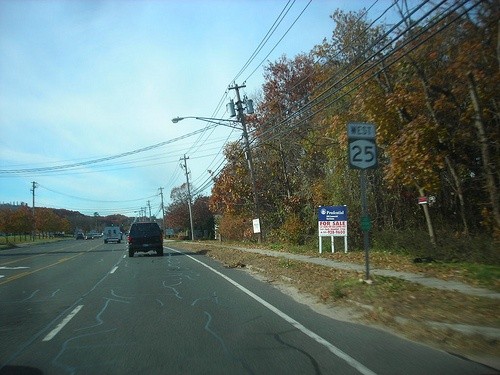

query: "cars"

left=74, top=230, right=129, bottom=241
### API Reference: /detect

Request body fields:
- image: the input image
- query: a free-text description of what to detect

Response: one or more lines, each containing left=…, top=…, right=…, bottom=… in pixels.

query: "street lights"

left=171, top=115, right=263, bottom=244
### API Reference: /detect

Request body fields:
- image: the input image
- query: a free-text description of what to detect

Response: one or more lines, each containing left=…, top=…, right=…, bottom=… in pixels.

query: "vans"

left=125, top=222, right=164, bottom=257
left=102, top=226, right=122, bottom=243
